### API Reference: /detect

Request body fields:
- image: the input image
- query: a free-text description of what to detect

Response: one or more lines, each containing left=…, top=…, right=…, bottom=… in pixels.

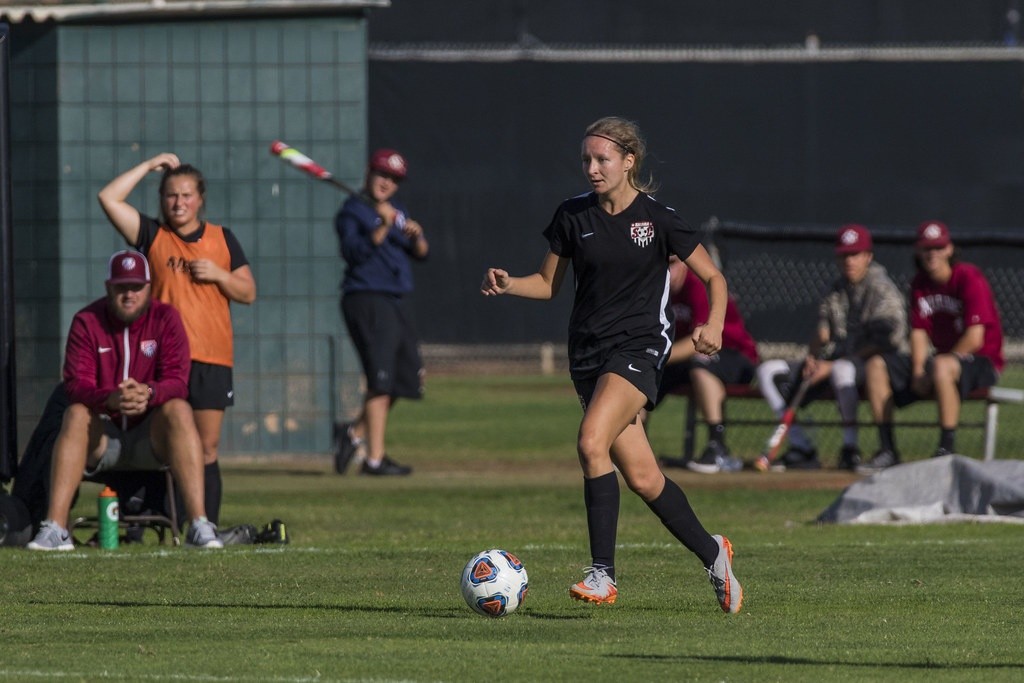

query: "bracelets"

left=148, top=384, right=154, bottom=404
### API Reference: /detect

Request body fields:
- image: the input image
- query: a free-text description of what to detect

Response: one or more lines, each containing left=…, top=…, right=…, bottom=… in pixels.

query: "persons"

left=481, top=116, right=743, bottom=615
left=98, top=152, right=256, bottom=545
left=27, top=250, right=224, bottom=552
left=334, top=149, right=428, bottom=477
left=756, top=224, right=905, bottom=471
left=856, top=220, right=1004, bottom=476
left=641, top=240, right=761, bottom=474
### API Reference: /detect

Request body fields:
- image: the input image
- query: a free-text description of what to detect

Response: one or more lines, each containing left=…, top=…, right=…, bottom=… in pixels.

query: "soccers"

left=460, top=549, right=530, bottom=618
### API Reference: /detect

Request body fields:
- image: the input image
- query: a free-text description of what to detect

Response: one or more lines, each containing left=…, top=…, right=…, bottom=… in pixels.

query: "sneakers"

left=253, top=520, right=290, bottom=545
left=331, top=421, right=363, bottom=478
left=218, top=523, right=259, bottom=546
left=686, top=445, right=732, bottom=474
left=932, top=447, right=958, bottom=459
left=834, top=443, right=862, bottom=468
left=701, top=534, right=744, bottom=617
left=355, top=456, right=416, bottom=480
left=28, top=519, right=77, bottom=553
left=183, top=519, right=225, bottom=552
left=867, top=444, right=903, bottom=469
left=568, top=563, right=619, bottom=606
left=781, top=445, right=824, bottom=469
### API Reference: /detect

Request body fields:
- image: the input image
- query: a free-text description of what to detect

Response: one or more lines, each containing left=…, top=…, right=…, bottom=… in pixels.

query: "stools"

left=68, top=464, right=181, bottom=547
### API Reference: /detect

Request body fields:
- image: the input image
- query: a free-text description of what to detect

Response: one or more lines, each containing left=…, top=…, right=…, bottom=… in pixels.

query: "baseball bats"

left=269, top=138, right=424, bottom=238
left=755, top=327, right=846, bottom=472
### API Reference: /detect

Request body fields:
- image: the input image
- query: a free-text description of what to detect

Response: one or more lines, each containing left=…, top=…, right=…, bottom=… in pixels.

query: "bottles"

left=98, top=486, right=120, bottom=551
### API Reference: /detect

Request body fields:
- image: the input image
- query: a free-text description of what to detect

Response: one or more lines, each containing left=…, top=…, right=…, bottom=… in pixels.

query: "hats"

left=832, top=222, right=874, bottom=255
left=370, top=149, right=409, bottom=180
left=109, top=249, right=153, bottom=287
left=913, top=220, right=951, bottom=252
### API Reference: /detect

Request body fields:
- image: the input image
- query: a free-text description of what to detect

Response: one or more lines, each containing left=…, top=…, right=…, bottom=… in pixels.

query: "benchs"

left=672, top=381, right=1024, bottom=464
